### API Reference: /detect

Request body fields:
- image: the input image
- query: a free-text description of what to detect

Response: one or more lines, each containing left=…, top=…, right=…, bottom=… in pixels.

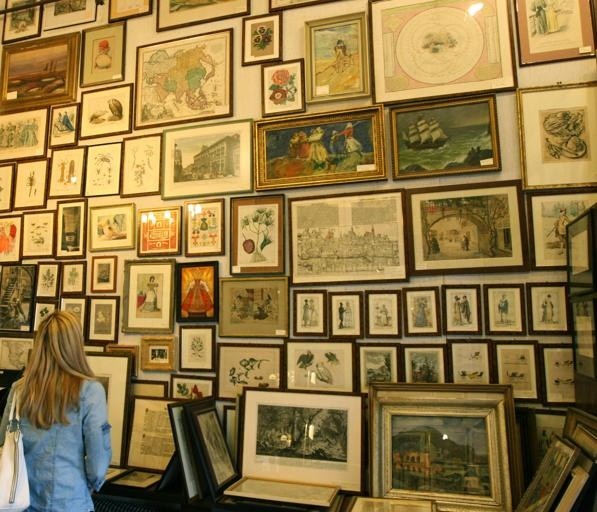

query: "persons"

left=0, top=311, right=112, bottom=512
left=553, top=207, right=571, bottom=254
left=302, top=299, right=310, bottom=328
left=308, top=299, right=319, bottom=327
left=540, top=293, right=555, bottom=323
left=288, top=122, right=363, bottom=170
left=338, top=302, right=352, bottom=329
left=0, top=119, right=38, bottom=148
left=463, top=235, right=469, bottom=251
left=453, top=295, right=473, bottom=327
left=530, top=0, right=547, bottom=34
left=53, top=111, right=74, bottom=133
left=497, top=293, right=510, bottom=322
left=138, top=275, right=160, bottom=313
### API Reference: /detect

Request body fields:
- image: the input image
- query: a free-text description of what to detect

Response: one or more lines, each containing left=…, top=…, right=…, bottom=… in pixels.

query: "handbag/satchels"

left=0, top=390, right=30, bottom=512
